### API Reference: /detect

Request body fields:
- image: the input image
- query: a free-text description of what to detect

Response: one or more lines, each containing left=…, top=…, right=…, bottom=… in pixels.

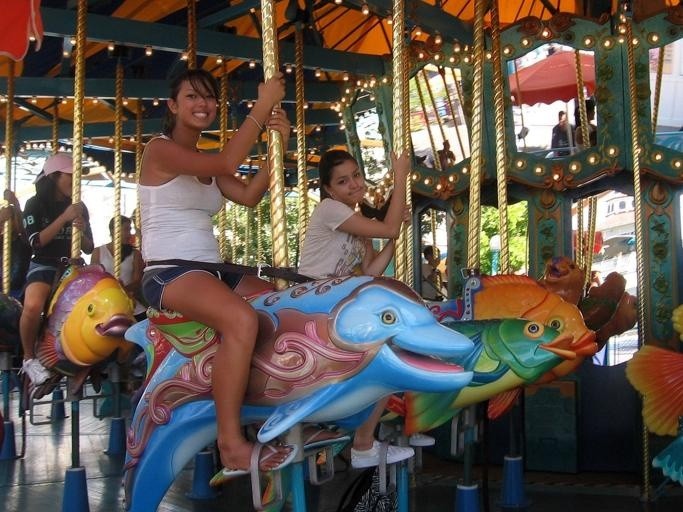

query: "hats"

left=42, top=153, right=73, bottom=176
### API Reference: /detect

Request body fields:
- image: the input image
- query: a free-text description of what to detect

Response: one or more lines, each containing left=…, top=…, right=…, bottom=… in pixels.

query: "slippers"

left=304, top=428, right=351, bottom=450
left=222, top=444, right=299, bottom=476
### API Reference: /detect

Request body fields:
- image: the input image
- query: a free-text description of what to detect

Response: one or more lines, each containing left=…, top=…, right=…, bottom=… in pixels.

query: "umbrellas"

left=509, top=44, right=596, bottom=108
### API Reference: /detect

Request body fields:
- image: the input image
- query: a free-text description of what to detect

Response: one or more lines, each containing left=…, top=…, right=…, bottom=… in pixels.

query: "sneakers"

left=351, top=440, right=415, bottom=469
left=22, top=359, right=51, bottom=385
left=398, top=432, right=436, bottom=446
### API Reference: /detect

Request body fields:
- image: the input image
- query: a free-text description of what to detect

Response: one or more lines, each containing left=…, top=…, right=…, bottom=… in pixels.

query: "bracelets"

left=246, top=113, right=264, bottom=131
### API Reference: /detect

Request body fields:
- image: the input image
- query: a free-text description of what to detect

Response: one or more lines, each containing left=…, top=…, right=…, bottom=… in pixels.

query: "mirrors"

left=417, top=205, right=449, bottom=305
left=478, top=200, right=530, bottom=278
left=506, top=42, right=597, bottom=162
left=353, top=107, right=391, bottom=184
left=407, top=64, right=471, bottom=173
left=570, top=191, right=641, bottom=366
left=648, top=38, right=683, bottom=156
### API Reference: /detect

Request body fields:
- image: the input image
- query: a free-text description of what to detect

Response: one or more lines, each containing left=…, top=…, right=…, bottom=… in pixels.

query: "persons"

left=18, top=154, right=93, bottom=385
left=90, top=214, right=143, bottom=291
left=431, top=139, right=456, bottom=170
left=129, top=210, right=142, bottom=249
left=574, top=99, right=596, bottom=150
left=0, top=187, right=32, bottom=301
left=421, top=244, right=445, bottom=302
left=294, top=148, right=437, bottom=470
left=137, top=68, right=352, bottom=475
left=552, top=111, right=575, bottom=157
